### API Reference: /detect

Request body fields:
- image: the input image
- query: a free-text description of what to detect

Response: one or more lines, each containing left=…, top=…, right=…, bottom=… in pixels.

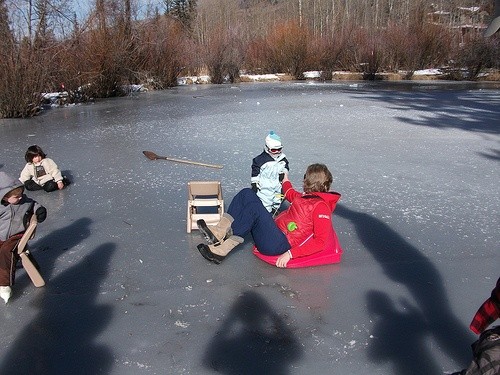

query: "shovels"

left=143, top=150, right=223, bottom=168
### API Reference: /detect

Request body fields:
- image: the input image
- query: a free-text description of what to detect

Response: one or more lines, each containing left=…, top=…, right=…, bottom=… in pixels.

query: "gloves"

left=36, top=204, right=47, bottom=223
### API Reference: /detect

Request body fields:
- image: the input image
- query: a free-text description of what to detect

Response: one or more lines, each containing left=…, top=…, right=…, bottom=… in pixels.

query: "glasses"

left=270, top=147, right=282, bottom=152
left=303, top=174, right=306, bottom=179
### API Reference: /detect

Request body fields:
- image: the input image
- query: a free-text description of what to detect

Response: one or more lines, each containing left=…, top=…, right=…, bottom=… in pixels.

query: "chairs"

left=186, top=181, right=225, bottom=234
left=17, top=214, right=46, bottom=288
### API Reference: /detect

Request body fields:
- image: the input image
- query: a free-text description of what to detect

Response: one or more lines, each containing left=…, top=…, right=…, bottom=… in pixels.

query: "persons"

left=251, top=130, right=290, bottom=216
left=0, top=171, right=47, bottom=303
left=19, top=145, right=70, bottom=192
left=196, top=164, right=341, bottom=269
left=448, top=276, right=500, bottom=375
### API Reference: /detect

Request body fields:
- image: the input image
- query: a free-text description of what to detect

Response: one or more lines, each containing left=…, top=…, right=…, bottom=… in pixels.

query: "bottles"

left=273, top=193, right=281, bottom=209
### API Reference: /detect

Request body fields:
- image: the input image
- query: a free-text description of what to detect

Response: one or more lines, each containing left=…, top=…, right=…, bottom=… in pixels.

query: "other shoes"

left=0, top=286, right=11, bottom=302
left=197, top=243, right=223, bottom=264
left=197, top=219, right=220, bottom=246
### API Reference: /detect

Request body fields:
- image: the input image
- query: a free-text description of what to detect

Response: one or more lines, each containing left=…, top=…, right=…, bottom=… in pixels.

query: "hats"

left=265, top=130, right=283, bottom=154
